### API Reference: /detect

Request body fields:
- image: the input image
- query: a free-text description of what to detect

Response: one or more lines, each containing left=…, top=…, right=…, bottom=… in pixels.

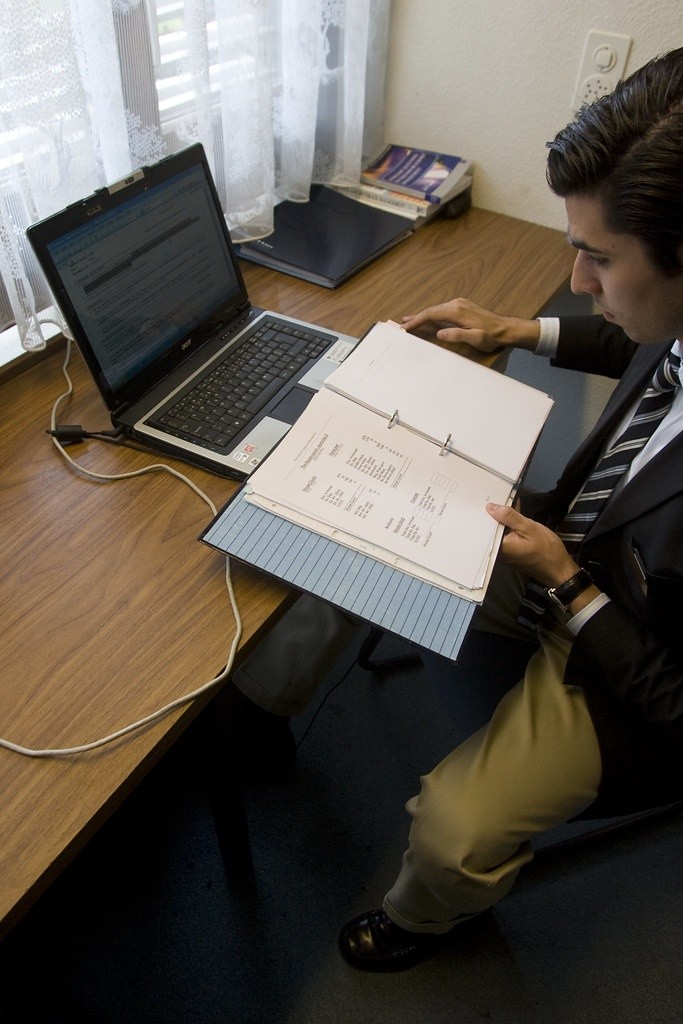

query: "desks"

left=1, top=205, right=580, bottom=933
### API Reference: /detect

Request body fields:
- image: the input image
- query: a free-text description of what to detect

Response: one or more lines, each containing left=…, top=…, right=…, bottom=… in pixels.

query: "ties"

left=514, top=346, right=683, bottom=646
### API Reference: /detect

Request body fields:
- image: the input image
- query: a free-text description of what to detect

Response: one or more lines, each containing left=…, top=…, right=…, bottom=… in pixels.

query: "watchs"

left=544, top=568, right=594, bottom=615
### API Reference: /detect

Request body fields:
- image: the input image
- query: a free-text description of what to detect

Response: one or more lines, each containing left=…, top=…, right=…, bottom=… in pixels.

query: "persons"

left=339, top=42, right=683, bottom=973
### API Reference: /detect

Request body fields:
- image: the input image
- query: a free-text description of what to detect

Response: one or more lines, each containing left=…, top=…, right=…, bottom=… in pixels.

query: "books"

left=331, top=144, right=474, bottom=230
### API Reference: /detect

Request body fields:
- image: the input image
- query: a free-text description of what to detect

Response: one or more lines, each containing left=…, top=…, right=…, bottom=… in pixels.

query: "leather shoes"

left=338, top=907, right=482, bottom=973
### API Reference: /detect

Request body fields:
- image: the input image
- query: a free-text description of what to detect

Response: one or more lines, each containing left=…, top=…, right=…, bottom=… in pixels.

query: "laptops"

left=26, top=142, right=360, bottom=482
